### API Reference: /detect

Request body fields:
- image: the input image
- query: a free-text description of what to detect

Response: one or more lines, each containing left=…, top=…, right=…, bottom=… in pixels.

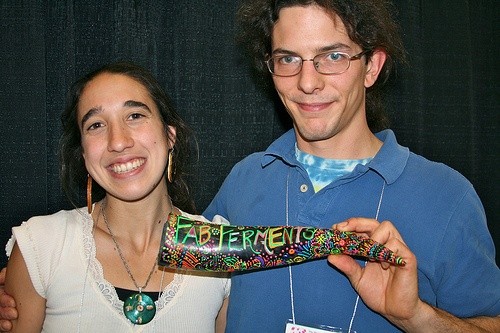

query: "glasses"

left=265, top=49, right=371, bottom=77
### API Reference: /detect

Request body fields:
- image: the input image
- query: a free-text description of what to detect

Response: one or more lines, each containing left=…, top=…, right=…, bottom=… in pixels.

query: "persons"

left=2, top=65, right=233, bottom=332
left=0, top=0, right=498, bottom=333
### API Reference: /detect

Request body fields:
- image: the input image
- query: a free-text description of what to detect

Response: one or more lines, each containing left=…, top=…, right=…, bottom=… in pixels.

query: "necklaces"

left=99, top=194, right=177, bottom=325
